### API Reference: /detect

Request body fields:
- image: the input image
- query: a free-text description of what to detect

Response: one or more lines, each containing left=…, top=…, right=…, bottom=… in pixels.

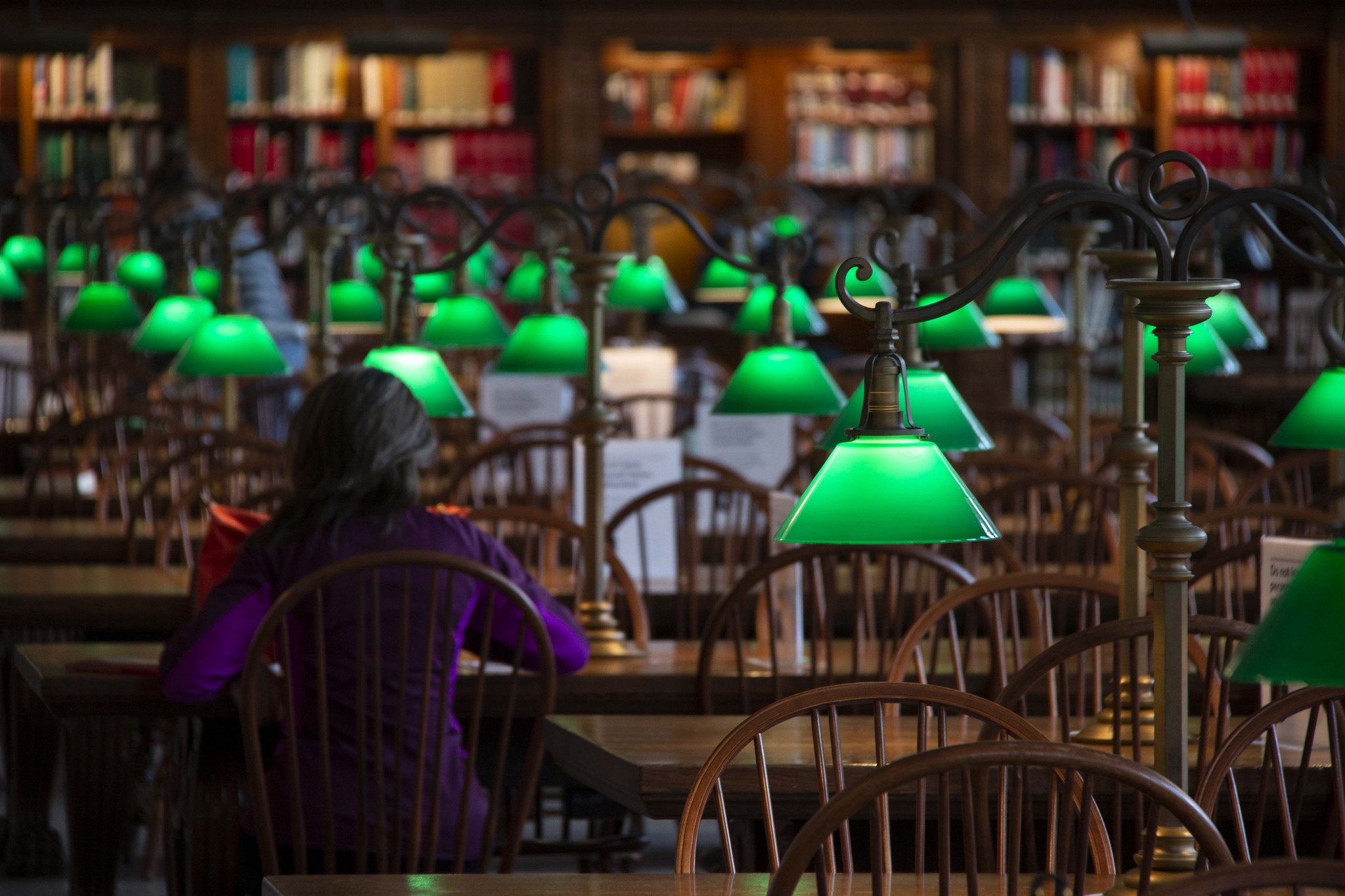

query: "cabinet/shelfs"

left=13, top=20, right=1345, bottom=226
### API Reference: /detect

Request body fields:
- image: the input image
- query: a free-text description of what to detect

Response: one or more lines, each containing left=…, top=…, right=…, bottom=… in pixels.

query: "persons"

left=153, top=366, right=589, bottom=870
left=141, top=135, right=304, bottom=441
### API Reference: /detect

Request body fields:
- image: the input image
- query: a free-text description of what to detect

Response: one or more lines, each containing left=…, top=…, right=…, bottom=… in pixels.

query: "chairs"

left=24, top=325, right=1345, bottom=896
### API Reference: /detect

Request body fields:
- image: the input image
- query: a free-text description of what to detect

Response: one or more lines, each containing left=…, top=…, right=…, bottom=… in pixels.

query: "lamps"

left=0, top=150, right=1345, bottom=895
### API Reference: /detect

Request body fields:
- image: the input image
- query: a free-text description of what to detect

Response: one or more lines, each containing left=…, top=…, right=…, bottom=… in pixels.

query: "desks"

left=545, top=711, right=1339, bottom=876
left=9, top=634, right=1249, bottom=892
left=0, top=559, right=1257, bottom=645
